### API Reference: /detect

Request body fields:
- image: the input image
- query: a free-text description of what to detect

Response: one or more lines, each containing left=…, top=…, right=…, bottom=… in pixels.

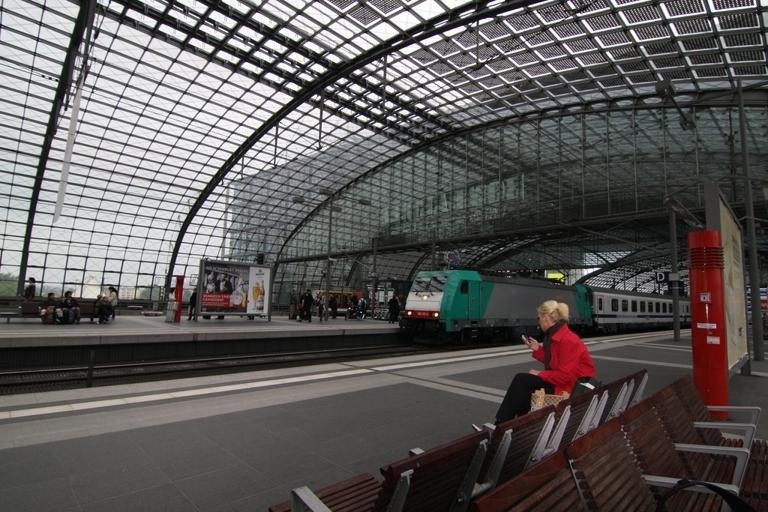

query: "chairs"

left=269, top=369, right=767, bottom=511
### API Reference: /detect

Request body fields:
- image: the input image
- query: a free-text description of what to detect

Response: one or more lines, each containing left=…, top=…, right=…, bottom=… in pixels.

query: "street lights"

left=422, top=231, right=440, bottom=271
left=292, top=188, right=371, bottom=321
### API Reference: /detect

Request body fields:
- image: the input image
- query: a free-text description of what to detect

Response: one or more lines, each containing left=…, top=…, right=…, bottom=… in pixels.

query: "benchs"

left=19, top=299, right=102, bottom=324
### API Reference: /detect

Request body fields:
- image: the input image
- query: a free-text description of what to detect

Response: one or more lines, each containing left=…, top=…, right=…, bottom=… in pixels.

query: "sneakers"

left=287, top=312, right=338, bottom=327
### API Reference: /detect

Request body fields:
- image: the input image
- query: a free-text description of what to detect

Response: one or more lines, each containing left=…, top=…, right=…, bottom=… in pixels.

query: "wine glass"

left=251, top=287, right=260, bottom=310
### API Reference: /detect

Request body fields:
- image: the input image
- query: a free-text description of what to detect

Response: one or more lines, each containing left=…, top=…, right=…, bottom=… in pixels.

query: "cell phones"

left=524, top=327, right=530, bottom=342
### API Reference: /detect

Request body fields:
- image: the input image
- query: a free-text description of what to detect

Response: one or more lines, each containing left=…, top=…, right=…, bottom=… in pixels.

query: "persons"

left=289, top=287, right=368, bottom=323
left=95, top=294, right=111, bottom=326
left=18, top=276, right=35, bottom=309
left=205, top=276, right=217, bottom=294
left=216, top=273, right=233, bottom=294
left=107, top=287, right=118, bottom=319
left=186, top=288, right=198, bottom=321
left=44, top=292, right=62, bottom=322
left=61, top=290, right=82, bottom=323
left=387, top=293, right=402, bottom=324
left=470, top=298, right=596, bottom=439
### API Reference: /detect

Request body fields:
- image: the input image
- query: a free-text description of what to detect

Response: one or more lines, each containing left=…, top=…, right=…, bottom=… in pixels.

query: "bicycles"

left=348, top=306, right=366, bottom=319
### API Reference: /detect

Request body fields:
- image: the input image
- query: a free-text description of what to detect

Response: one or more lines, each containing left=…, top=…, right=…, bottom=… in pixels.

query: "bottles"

left=255, top=280, right=265, bottom=311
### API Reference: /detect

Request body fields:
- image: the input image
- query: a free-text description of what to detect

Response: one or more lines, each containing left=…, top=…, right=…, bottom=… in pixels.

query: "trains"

left=399, top=270, right=691, bottom=352
left=358, top=275, right=413, bottom=305
